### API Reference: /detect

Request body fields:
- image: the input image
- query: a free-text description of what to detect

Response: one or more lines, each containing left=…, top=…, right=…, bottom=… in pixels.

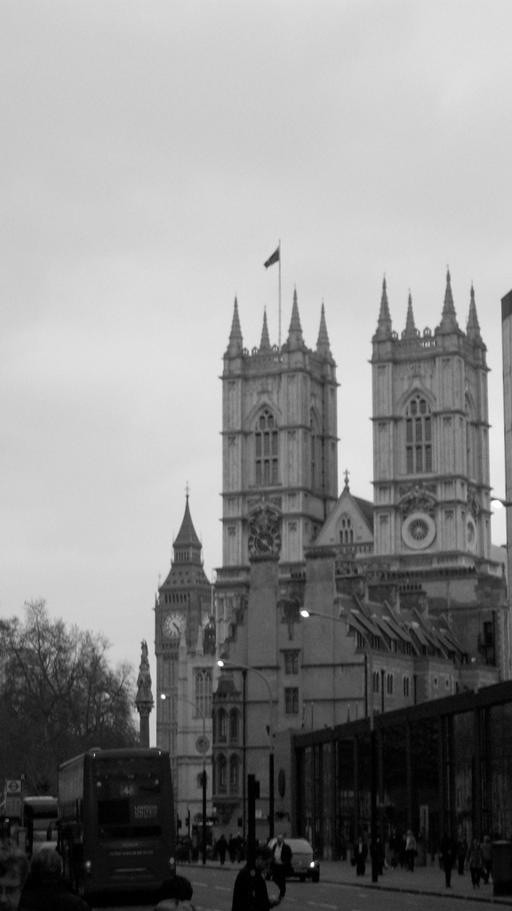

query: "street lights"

left=160, top=693, right=208, bottom=868
left=215, top=657, right=278, bottom=866
left=298, top=608, right=384, bottom=884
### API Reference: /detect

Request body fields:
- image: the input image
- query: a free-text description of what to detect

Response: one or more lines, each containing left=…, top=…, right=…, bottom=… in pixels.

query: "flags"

left=264, top=246, right=280, bottom=270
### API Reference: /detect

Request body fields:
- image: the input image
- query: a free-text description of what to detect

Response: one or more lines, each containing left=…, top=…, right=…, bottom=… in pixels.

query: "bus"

left=2, top=795, right=62, bottom=841
left=44, top=748, right=181, bottom=908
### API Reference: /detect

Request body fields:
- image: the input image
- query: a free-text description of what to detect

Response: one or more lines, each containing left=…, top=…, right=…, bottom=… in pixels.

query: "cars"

left=262, top=836, right=321, bottom=885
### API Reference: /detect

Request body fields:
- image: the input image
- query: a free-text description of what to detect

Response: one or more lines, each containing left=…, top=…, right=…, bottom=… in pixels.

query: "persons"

left=1, top=820, right=27, bottom=911
left=269, top=833, right=292, bottom=899
left=180, top=835, right=246, bottom=866
left=231, top=843, right=279, bottom=911
left=349, top=822, right=492, bottom=891
left=20, top=846, right=87, bottom=910
